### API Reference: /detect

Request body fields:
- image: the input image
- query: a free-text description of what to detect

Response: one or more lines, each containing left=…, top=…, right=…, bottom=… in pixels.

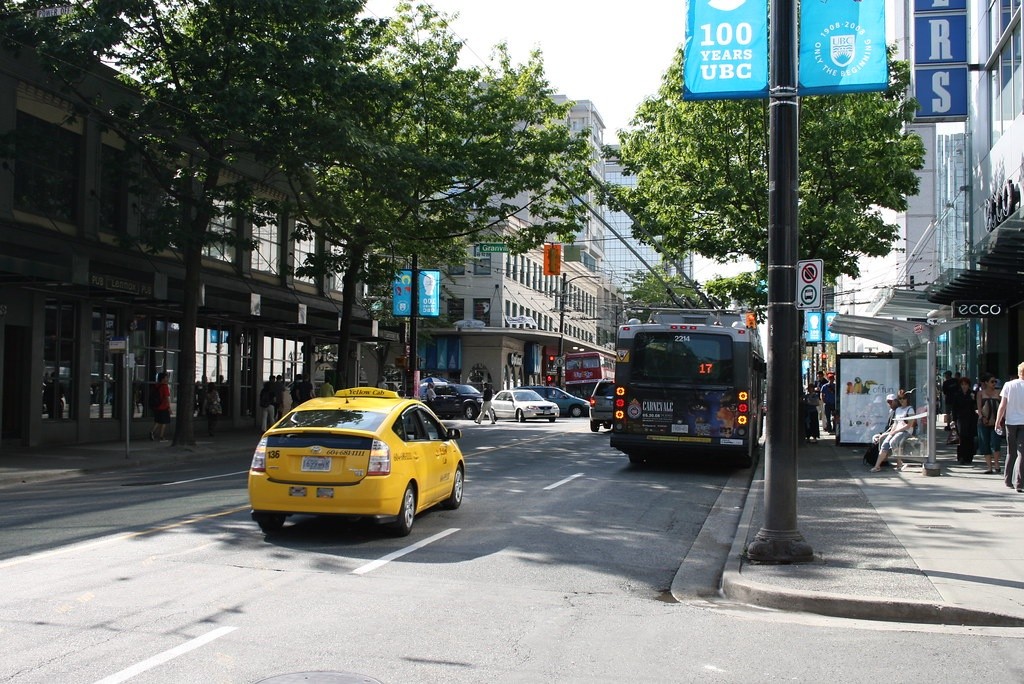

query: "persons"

left=261, top=374, right=316, bottom=432
left=820, top=374, right=836, bottom=435
left=869, top=390, right=916, bottom=473
left=941, top=370, right=981, bottom=463
left=425, top=383, right=437, bottom=407
left=203, top=383, right=222, bottom=437
left=42, top=373, right=66, bottom=417
left=814, top=370, right=828, bottom=429
left=377, top=375, right=389, bottom=390
left=995, top=362, right=1024, bottom=492
left=149, top=372, right=174, bottom=442
left=319, top=376, right=336, bottom=396
left=802, top=383, right=821, bottom=442
left=474, top=382, right=496, bottom=424
left=973, top=373, right=1005, bottom=473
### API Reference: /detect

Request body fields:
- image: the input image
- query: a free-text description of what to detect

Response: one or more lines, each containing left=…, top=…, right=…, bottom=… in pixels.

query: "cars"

left=246, top=386, right=466, bottom=536
left=589, top=380, right=615, bottom=432
left=489, top=386, right=590, bottom=423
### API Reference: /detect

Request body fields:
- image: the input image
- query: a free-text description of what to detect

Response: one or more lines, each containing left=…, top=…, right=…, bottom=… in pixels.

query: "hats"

left=827, top=374, right=834, bottom=379
left=886, top=394, right=898, bottom=401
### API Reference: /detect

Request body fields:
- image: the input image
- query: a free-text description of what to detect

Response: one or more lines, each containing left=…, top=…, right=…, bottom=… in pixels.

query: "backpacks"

left=149, top=384, right=161, bottom=409
left=260, top=391, right=270, bottom=408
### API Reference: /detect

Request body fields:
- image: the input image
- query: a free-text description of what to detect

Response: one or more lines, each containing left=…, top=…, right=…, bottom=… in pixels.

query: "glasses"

left=990, top=380, right=997, bottom=383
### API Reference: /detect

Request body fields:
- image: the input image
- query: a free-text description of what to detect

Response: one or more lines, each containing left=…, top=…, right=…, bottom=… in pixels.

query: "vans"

left=418, top=384, right=489, bottom=419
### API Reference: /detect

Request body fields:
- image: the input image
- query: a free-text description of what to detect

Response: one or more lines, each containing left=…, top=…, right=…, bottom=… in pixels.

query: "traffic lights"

left=819, top=354, right=827, bottom=367
left=546, top=375, right=555, bottom=383
left=746, top=313, right=756, bottom=330
left=549, top=355, right=555, bottom=368
left=813, top=354, right=818, bottom=372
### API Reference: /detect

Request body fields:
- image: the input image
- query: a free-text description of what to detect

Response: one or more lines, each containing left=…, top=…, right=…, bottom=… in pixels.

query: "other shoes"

left=871, top=467, right=881, bottom=472
left=150, top=431, right=155, bottom=441
left=897, top=460, right=903, bottom=470
left=984, top=470, right=993, bottom=474
left=159, top=437, right=168, bottom=443
left=995, top=468, right=1001, bottom=472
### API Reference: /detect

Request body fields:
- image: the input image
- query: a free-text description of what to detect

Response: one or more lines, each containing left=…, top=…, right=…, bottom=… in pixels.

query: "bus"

left=610, top=288, right=767, bottom=470
left=565, top=351, right=615, bottom=400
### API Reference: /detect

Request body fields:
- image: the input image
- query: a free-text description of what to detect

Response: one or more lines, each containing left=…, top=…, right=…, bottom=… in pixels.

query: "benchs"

left=906, top=437, right=921, bottom=446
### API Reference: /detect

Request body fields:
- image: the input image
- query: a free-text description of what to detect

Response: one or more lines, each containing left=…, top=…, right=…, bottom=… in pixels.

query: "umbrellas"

left=420, top=376, right=448, bottom=382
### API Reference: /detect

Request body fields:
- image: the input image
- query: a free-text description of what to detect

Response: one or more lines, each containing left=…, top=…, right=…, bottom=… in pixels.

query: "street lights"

left=555, top=273, right=601, bottom=388
left=614, top=306, right=632, bottom=350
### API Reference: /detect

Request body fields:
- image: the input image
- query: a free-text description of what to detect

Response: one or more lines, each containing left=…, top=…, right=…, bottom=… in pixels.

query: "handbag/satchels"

left=946, top=428, right=960, bottom=445
left=863, top=444, right=879, bottom=465
